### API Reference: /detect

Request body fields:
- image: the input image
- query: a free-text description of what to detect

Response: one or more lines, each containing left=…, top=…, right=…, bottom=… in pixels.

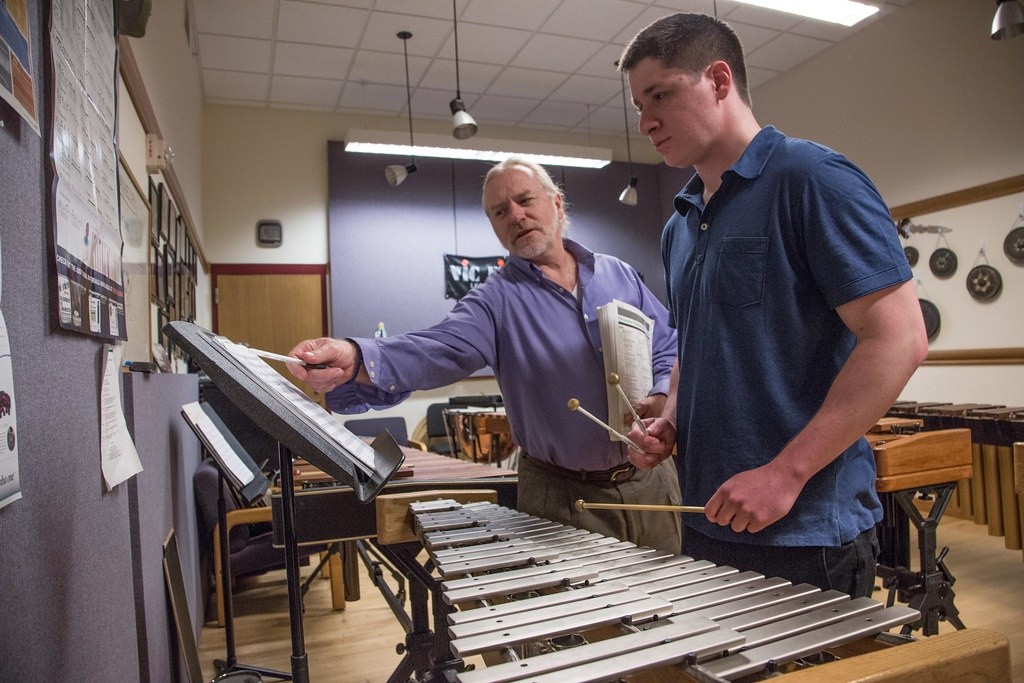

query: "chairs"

left=194, top=460, right=346, bottom=629
left=344, top=416, right=428, bottom=453
left=426, top=396, right=519, bottom=470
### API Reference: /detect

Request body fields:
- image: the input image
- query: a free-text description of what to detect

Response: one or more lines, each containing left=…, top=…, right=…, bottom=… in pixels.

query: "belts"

left=523, top=452, right=639, bottom=488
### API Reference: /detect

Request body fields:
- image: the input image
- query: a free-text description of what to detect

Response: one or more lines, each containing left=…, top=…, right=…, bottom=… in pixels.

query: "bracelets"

left=345, top=338, right=363, bottom=385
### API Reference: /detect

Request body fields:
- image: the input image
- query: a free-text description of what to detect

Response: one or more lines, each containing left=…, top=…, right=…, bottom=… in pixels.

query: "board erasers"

left=132, top=361, right=159, bottom=373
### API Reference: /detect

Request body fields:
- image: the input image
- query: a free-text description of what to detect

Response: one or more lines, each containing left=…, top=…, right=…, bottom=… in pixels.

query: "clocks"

left=258, top=222, right=283, bottom=249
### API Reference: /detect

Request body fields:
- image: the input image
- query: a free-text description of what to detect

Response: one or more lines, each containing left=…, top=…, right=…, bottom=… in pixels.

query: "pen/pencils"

left=254, top=347, right=330, bottom=368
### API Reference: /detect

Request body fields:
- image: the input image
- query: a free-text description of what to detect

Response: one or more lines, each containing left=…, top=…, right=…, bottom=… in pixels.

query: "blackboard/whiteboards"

left=100, top=148, right=152, bottom=368
left=321, top=137, right=702, bottom=378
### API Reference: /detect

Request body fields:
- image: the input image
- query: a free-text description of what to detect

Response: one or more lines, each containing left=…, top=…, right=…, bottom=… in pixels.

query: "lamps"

left=990, top=0, right=1024, bottom=41
left=450, top=1, right=478, bottom=141
left=615, top=59, right=639, bottom=207
left=385, top=31, right=417, bottom=187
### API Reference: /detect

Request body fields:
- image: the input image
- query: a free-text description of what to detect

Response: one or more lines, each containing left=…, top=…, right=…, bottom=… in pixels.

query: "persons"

left=619, top=13, right=929, bottom=594
left=286, top=157, right=682, bottom=555
